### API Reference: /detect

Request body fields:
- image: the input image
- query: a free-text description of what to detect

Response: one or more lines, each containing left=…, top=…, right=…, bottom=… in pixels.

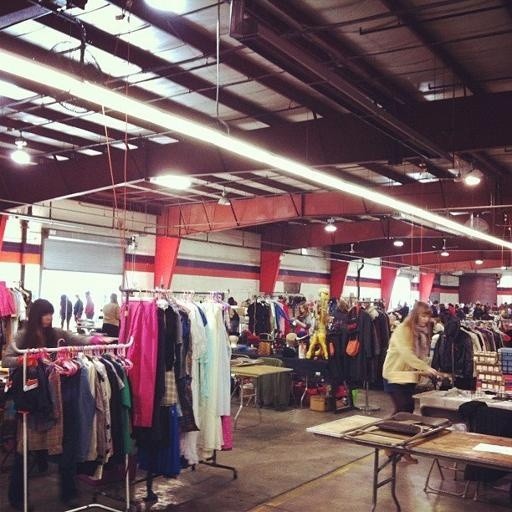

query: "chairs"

left=339, top=411, right=452, bottom=447
left=420, top=407, right=469, bottom=500
left=255, top=356, right=297, bottom=408
left=472, top=406, right=512, bottom=506
left=231, top=355, right=251, bottom=406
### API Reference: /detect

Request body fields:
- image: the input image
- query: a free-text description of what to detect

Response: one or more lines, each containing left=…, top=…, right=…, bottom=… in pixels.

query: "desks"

left=306, top=412, right=512, bottom=511
left=285, top=358, right=330, bottom=408
left=231, top=365, right=294, bottom=430
left=413, top=391, right=512, bottom=420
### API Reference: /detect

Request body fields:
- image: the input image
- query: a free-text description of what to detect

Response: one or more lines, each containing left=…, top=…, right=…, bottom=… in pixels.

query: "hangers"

left=56, top=339, right=65, bottom=347
left=38, top=347, right=40, bottom=353
left=27, top=348, right=30, bottom=355
left=42, top=344, right=134, bottom=375
left=32, top=348, right=34, bottom=353
left=138, top=290, right=231, bottom=311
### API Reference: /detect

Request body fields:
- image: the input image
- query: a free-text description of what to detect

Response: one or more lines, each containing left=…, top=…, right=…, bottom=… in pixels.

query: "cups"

left=453, top=423, right=467, bottom=438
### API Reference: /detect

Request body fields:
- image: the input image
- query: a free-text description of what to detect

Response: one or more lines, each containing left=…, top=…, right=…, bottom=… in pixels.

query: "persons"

left=431, top=300, right=438, bottom=318
left=59, top=295, right=72, bottom=331
left=448, top=300, right=512, bottom=321
left=382, top=301, right=439, bottom=415
left=102, top=293, right=123, bottom=337
left=2, top=298, right=115, bottom=368
left=85, top=291, right=95, bottom=319
left=74, top=294, right=84, bottom=333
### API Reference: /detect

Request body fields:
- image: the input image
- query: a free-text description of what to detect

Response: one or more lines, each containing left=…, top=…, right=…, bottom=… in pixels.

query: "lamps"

left=217, top=190, right=231, bottom=205
